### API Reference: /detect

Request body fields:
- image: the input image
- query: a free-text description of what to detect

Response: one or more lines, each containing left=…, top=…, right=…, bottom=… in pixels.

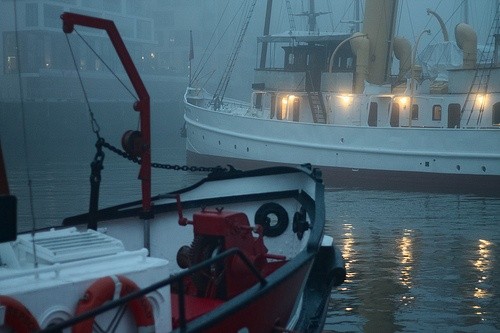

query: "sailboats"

left=174, top=3, right=499, bottom=195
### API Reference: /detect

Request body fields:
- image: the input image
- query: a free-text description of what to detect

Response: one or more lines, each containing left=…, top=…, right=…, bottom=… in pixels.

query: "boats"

left=0, top=11, right=348, bottom=333
